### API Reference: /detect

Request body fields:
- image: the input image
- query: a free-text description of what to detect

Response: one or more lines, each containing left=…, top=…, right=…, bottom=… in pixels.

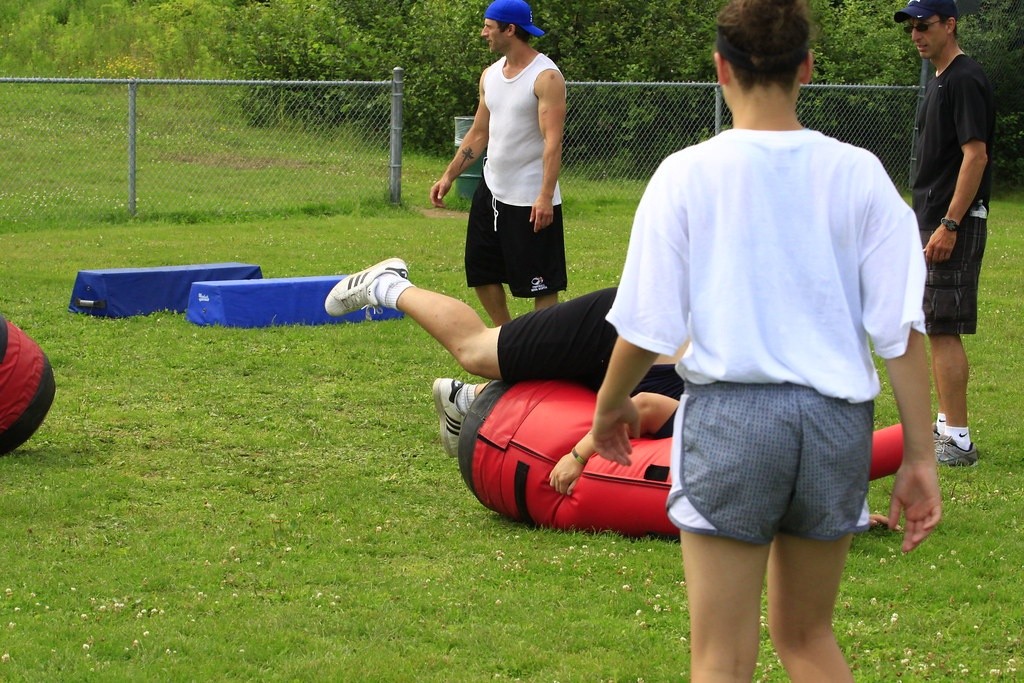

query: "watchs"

left=571, top=447, right=588, bottom=465
left=940, top=218, right=960, bottom=233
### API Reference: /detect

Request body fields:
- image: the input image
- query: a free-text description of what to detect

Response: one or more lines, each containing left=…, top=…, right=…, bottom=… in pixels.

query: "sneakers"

left=432, top=375, right=466, bottom=458
left=324, top=256, right=407, bottom=317
left=929, top=422, right=979, bottom=467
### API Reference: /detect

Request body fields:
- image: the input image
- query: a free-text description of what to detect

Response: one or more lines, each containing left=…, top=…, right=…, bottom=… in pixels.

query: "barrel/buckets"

left=454, top=116, right=488, bottom=198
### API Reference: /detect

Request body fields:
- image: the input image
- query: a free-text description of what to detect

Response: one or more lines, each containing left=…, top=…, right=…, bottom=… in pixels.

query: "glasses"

left=903, top=19, right=940, bottom=32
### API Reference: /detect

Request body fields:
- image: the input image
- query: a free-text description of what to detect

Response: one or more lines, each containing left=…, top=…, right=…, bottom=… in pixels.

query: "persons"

left=591, top=0, right=940, bottom=683
left=325, top=256, right=901, bottom=533
left=895, top=0, right=998, bottom=467
left=429, top=0, right=568, bottom=328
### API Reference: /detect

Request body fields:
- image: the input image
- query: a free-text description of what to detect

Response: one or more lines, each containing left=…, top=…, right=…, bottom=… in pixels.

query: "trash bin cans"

left=453, top=116, right=488, bottom=200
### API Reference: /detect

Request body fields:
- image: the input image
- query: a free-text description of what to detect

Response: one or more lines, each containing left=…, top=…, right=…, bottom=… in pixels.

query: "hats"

left=483, top=1, right=548, bottom=37
left=893, top=0, right=961, bottom=24
